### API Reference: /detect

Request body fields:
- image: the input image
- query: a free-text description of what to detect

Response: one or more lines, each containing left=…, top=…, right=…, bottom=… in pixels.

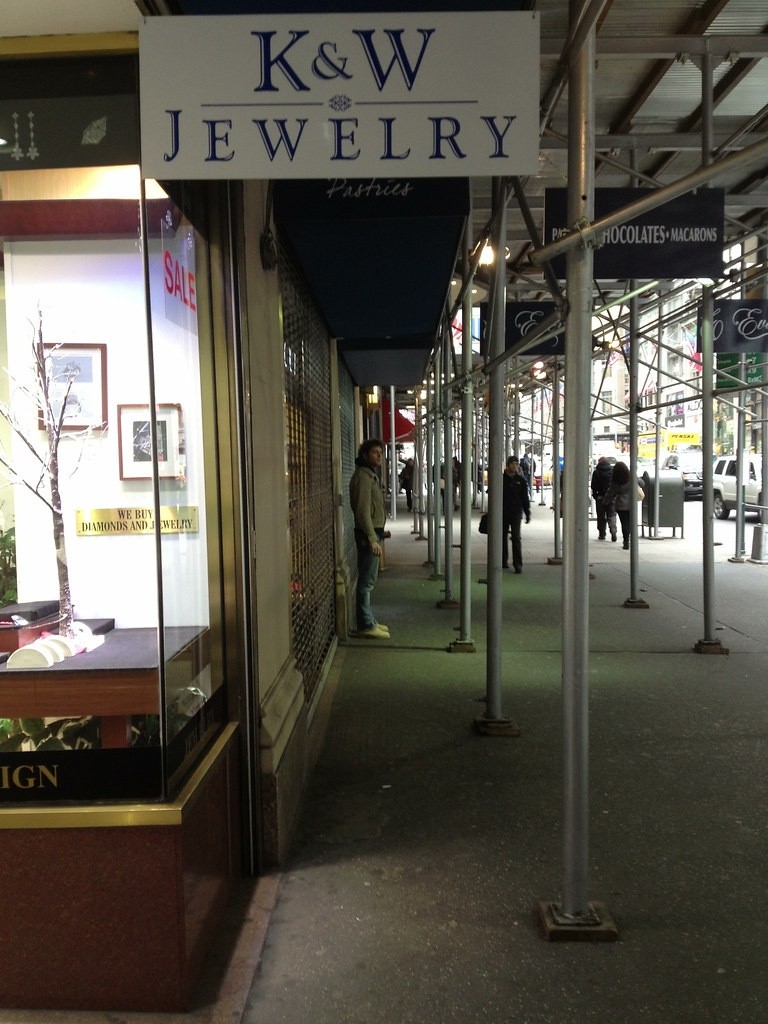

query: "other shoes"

left=598, top=536, right=605, bottom=539
left=359, top=622, right=391, bottom=639
left=409, top=509, right=412, bottom=512
left=623, top=545, right=629, bottom=549
left=612, top=534, right=616, bottom=542
left=455, top=505, right=460, bottom=511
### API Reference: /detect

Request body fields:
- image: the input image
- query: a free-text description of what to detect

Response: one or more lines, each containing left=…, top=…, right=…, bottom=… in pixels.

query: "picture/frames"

left=31, top=339, right=111, bottom=432
left=117, top=403, right=190, bottom=480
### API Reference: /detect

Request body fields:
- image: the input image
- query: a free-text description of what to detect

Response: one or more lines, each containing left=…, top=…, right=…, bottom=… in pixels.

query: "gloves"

left=525, top=513, right=531, bottom=523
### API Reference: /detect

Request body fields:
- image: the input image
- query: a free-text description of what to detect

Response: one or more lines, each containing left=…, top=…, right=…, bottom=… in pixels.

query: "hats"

left=508, top=456, right=518, bottom=462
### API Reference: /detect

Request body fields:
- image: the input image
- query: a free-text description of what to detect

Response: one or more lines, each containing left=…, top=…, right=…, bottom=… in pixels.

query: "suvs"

left=662, top=450, right=714, bottom=501
left=711, top=455, right=766, bottom=524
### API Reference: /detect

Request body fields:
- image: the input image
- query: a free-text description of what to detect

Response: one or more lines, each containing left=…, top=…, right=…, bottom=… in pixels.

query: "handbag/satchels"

left=479, top=515, right=488, bottom=534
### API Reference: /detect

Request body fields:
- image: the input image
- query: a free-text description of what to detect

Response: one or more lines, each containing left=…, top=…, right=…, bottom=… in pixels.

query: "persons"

left=398, top=458, right=415, bottom=511
left=348, top=439, right=391, bottom=639
left=501, top=456, right=532, bottom=573
left=591, top=457, right=619, bottom=542
left=520, top=454, right=536, bottom=499
left=602, top=462, right=645, bottom=550
left=431, top=456, right=484, bottom=512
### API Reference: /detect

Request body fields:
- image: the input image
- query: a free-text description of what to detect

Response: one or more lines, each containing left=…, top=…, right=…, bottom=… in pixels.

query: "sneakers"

left=515, top=566, right=522, bottom=573
left=503, top=562, right=509, bottom=568
